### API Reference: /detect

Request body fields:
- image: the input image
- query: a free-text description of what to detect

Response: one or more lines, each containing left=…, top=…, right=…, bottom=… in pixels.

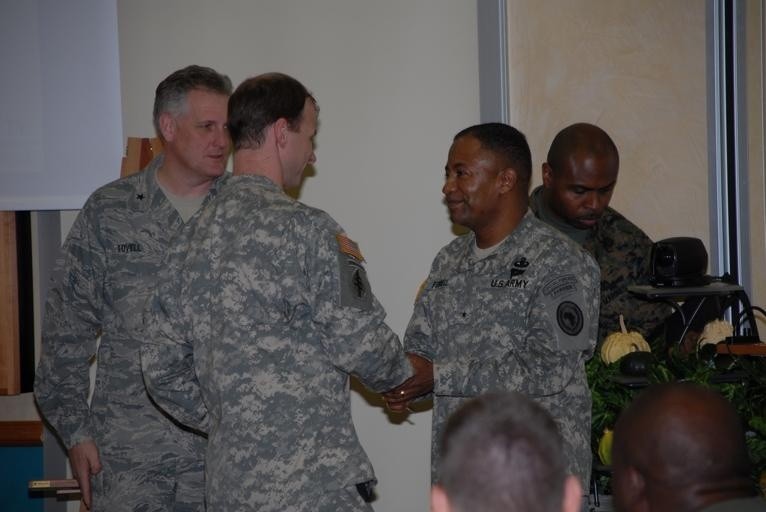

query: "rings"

left=401, top=391, right=404, bottom=398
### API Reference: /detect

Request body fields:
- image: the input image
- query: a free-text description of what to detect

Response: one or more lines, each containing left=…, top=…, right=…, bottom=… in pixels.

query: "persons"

left=429, top=389, right=583, bottom=512
left=139, top=71, right=414, bottom=511
left=33, top=65, right=234, bottom=511
left=379, top=121, right=602, bottom=512
left=526, top=122, right=671, bottom=341
left=610, top=381, right=766, bottom=512
